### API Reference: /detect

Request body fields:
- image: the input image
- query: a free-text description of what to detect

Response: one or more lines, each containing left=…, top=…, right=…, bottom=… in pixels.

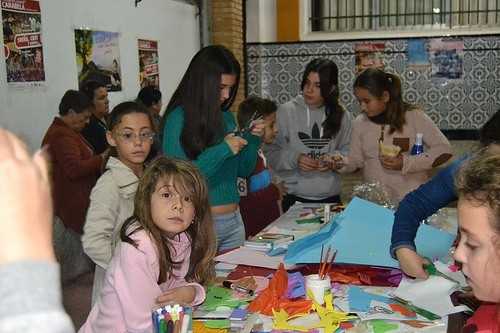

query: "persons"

left=325, top=66, right=453, bottom=208
left=136, top=85, right=164, bottom=134
left=157, top=44, right=265, bottom=256
left=40, top=88, right=110, bottom=235
left=80, top=80, right=121, bottom=181
left=235, top=97, right=289, bottom=237
left=389, top=111, right=499, bottom=333
left=80, top=101, right=157, bottom=309
left=263, top=57, right=354, bottom=212
left=75, top=157, right=218, bottom=333
left=1, top=124, right=76, bottom=333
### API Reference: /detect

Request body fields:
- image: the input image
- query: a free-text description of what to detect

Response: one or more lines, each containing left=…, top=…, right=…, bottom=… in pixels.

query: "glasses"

left=113, top=129, right=156, bottom=142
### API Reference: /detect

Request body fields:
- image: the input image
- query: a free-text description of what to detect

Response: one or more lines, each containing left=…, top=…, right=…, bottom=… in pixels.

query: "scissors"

left=320, top=159, right=346, bottom=165
left=422, top=257, right=460, bottom=284
left=228, top=110, right=264, bottom=139
left=299, top=208, right=318, bottom=217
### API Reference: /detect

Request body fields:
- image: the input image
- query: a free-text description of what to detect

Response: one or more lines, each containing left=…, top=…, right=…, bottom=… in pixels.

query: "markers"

left=150, top=304, right=193, bottom=333
left=223, top=281, right=254, bottom=296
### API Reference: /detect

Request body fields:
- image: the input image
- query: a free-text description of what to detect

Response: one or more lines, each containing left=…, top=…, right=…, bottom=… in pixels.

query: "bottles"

left=410, top=133, right=424, bottom=156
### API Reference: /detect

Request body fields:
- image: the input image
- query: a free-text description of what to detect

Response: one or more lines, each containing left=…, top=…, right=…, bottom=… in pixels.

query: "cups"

left=150, top=301, right=194, bottom=333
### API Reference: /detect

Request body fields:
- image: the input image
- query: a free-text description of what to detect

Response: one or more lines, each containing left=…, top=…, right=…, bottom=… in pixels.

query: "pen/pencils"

left=214, top=268, right=234, bottom=273
left=302, top=207, right=322, bottom=209
left=388, top=293, right=413, bottom=306
left=319, top=244, right=338, bottom=280
